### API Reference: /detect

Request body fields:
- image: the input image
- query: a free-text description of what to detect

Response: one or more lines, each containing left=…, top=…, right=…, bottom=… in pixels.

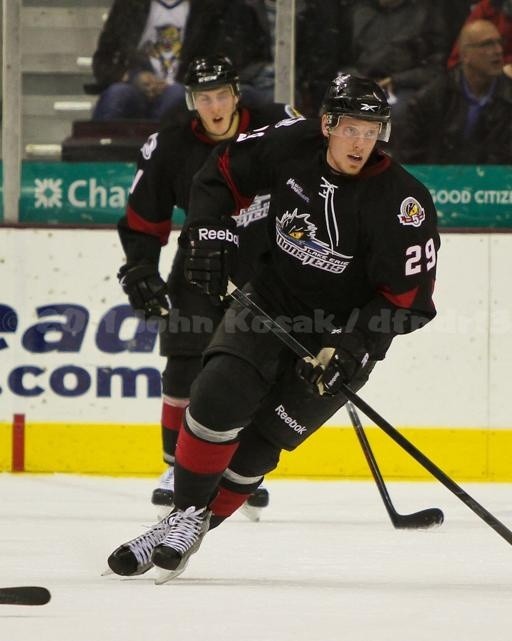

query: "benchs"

left=59, top=113, right=164, bottom=164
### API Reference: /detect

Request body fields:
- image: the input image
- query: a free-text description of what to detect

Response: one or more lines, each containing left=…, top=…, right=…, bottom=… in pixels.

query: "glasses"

left=465, top=38, right=505, bottom=49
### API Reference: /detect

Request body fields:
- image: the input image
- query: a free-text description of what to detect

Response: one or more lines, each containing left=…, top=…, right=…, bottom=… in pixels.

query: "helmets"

left=183, top=56, right=241, bottom=112
left=318, top=73, right=392, bottom=143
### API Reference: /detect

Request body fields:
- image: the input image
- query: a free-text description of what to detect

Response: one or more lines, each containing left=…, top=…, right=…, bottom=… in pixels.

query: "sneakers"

left=150, top=465, right=174, bottom=505
left=150, top=506, right=212, bottom=570
left=108, top=508, right=182, bottom=576
left=246, top=481, right=269, bottom=507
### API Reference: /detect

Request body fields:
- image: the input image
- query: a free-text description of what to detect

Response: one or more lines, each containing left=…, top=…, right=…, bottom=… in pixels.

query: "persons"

left=116, top=52, right=303, bottom=508
left=107, top=72, right=442, bottom=576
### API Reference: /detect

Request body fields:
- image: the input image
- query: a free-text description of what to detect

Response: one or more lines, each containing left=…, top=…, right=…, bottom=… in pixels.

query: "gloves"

left=116, top=263, right=171, bottom=321
left=297, top=331, right=368, bottom=399
left=177, top=224, right=231, bottom=306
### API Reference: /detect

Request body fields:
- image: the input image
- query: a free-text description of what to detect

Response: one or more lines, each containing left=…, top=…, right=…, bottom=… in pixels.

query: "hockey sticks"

left=346, top=400, right=444, bottom=528
